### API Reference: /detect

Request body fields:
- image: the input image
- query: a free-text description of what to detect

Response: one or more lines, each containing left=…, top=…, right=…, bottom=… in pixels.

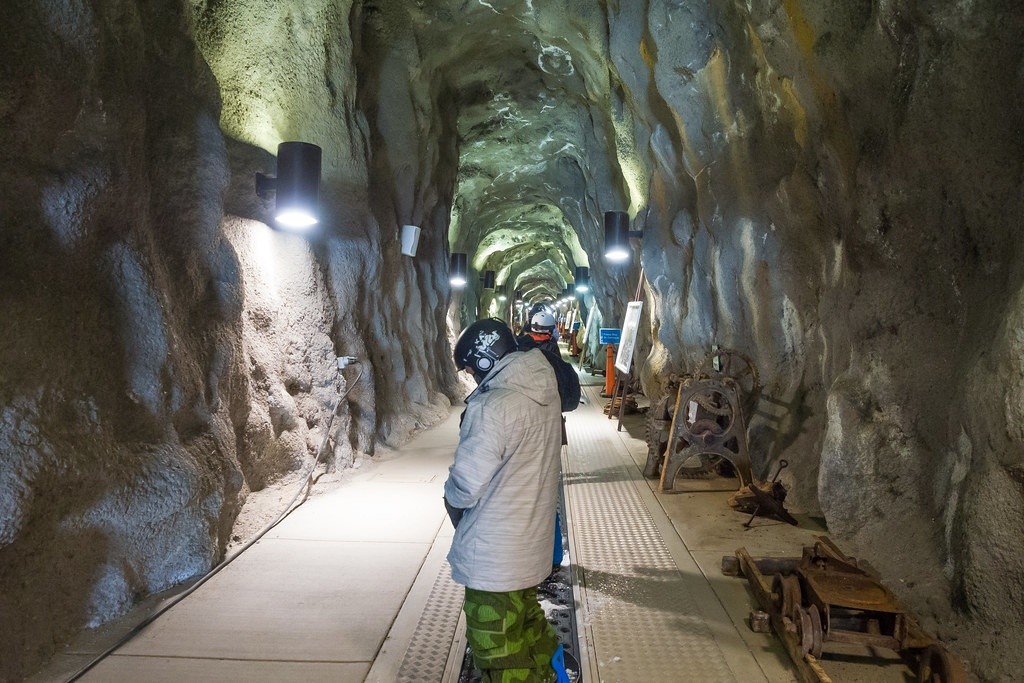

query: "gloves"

left=444, top=496, right=464, bottom=529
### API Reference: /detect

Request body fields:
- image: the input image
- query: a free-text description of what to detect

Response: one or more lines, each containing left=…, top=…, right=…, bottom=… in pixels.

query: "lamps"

left=528, top=302, right=532, bottom=310
left=575, top=266, right=590, bottom=292
left=524, top=297, right=529, bottom=306
left=254, top=140, right=323, bottom=230
left=515, top=291, right=523, bottom=302
left=479, top=270, right=495, bottom=290
left=495, top=284, right=506, bottom=301
left=567, top=283, right=575, bottom=300
left=549, top=288, right=567, bottom=310
left=603, top=209, right=643, bottom=260
left=449, top=253, right=468, bottom=285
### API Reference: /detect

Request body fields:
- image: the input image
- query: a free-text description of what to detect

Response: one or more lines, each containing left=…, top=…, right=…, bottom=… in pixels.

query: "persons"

left=444, top=304, right=581, bottom=683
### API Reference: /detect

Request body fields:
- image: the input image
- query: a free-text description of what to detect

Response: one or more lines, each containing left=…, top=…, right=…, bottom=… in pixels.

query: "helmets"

left=531, top=311, right=556, bottom=335
left=534, top=303, right=545, bottom=311
left=454, top=318, right=516, bottom=386
